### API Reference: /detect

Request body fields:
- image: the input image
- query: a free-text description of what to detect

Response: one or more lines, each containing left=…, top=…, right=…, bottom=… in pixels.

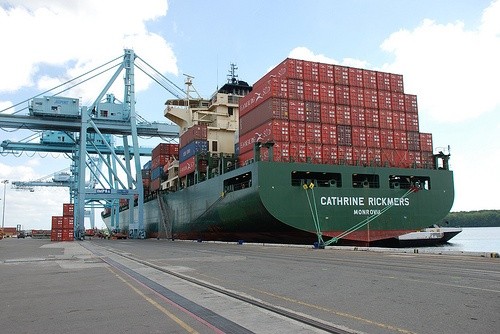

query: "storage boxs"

left=239, top=58, right=436, bottom=170
left=102, top=124, right=209, bottom=213
left=51, top=204, right=74, bottom=241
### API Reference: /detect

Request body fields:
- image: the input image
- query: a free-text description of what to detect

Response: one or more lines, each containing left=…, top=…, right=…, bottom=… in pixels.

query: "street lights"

left=2, top=179, right=10, bottom=230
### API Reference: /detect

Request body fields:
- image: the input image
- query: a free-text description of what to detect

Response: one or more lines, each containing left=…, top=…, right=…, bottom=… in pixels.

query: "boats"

left=370, top=228, right=462, bottom=248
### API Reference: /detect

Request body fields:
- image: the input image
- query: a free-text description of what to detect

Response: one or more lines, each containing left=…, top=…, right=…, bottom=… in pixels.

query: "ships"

left=99, top=62, right=455, bottom=244
